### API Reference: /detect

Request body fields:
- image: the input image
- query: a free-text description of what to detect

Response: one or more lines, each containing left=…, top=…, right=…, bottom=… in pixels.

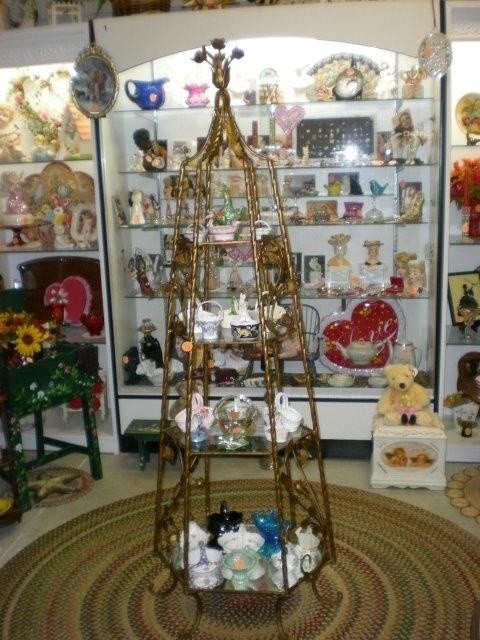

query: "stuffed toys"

left=374, top=363, right=433, bottom=425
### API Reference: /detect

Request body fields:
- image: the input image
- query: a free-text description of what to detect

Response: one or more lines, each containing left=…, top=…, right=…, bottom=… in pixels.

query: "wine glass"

left=286, top=184, right=306, bottom=226
left=363, top=196, right=384, bottom=221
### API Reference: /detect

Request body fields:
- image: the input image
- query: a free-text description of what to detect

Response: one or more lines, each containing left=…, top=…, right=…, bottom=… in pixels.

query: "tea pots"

left=325, top=371, right=357, bottom=387
left=334, top=338, right=385, bottom=366
left=386, top=337, right=425, bottom=373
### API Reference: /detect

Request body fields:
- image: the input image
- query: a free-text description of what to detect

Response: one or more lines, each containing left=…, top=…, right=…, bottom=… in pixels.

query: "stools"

left=370, top=414, right=448, bottom=492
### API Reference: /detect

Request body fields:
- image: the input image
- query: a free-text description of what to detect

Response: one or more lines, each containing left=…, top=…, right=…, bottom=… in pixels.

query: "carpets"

left=445, top=465, right=480, bottom=526
left=0, top=477, right=480, bottom=640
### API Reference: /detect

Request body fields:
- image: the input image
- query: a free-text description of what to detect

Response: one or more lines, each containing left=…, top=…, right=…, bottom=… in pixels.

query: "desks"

left=0, top=341, right=104, bottom=512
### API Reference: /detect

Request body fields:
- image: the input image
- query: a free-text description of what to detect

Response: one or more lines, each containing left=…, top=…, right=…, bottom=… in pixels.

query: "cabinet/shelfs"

left=145, top=37, right=343, bottom=640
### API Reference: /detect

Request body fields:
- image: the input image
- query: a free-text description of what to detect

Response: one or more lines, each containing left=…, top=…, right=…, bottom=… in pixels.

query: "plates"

left=455, top=92, right=480, bottom=139
left=335, top=66, right=364, bottom=99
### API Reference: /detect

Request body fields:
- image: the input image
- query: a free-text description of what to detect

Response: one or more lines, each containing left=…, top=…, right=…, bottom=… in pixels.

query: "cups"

left=367, top=369, right=389, bottom=388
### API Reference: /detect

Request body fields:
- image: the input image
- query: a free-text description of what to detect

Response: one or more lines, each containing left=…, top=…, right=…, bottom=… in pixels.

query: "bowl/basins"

left=146, top=367, right=175, bottom=387
